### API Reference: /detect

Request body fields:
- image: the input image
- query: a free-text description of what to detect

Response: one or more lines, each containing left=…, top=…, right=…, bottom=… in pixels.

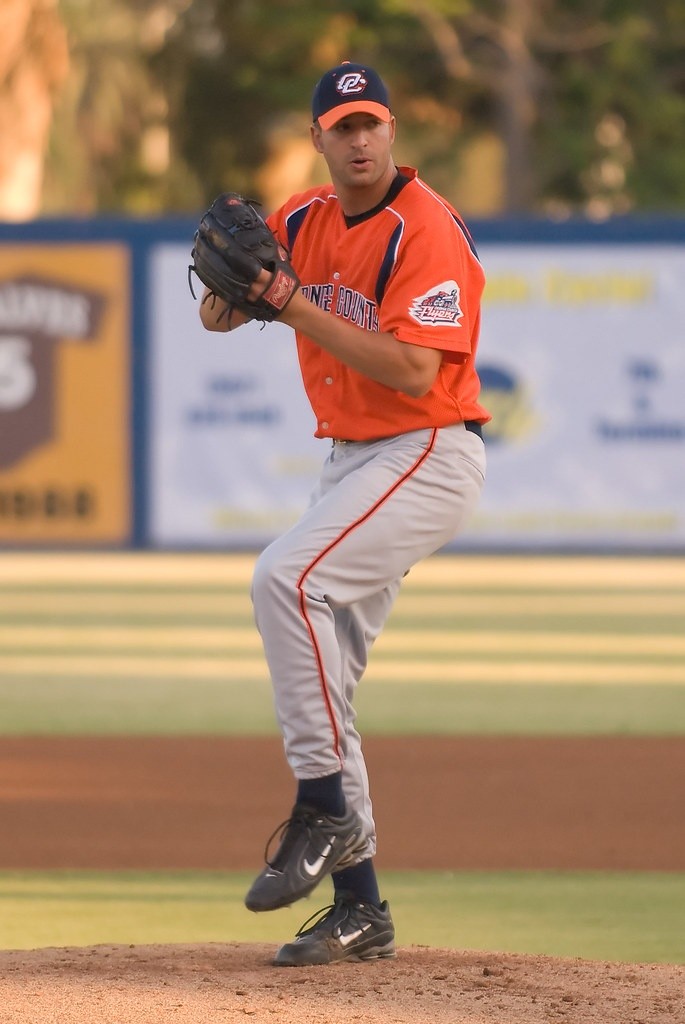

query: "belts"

left=338, top=421, right=484, bottom=445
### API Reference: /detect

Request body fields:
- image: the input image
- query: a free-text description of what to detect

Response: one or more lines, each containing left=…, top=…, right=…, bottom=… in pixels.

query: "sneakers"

left=244, top=795, right=373, bottom=913
left=273, top=899, right=396, bottom=967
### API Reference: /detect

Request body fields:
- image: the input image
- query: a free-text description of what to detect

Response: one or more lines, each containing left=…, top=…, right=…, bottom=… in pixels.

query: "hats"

left=312, top=61, right=392, bottom=128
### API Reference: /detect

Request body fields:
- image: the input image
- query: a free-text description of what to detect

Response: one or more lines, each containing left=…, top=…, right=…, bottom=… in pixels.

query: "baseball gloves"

left=183, top=188, right=303, bottom=331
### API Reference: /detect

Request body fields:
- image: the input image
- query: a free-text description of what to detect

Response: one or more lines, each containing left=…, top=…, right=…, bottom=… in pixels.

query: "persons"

left=188, top=61, right=493, bottom=967
